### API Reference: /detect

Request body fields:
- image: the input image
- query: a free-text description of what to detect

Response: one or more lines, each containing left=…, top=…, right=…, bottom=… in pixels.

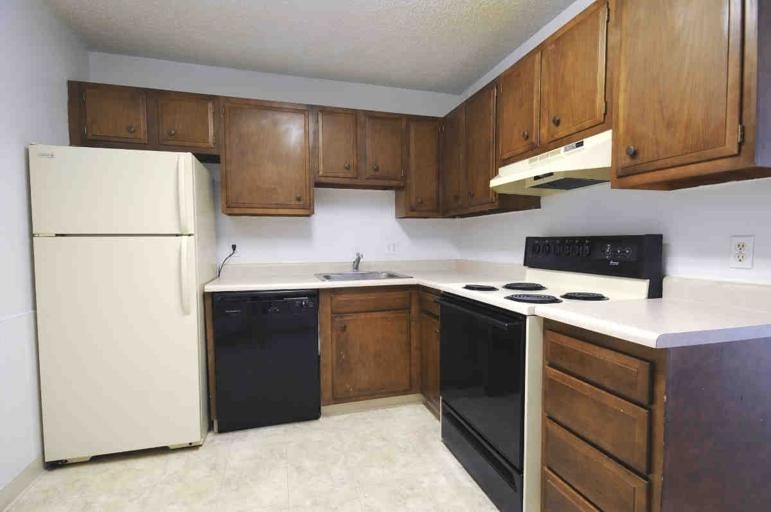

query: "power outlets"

left=226, top=242, right=239, bottom=256
left=730, top=237, right=752, bottom=268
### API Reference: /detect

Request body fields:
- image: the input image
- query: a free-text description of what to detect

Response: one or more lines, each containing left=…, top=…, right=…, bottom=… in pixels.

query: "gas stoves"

left=438, top=280, right=645, bottom=307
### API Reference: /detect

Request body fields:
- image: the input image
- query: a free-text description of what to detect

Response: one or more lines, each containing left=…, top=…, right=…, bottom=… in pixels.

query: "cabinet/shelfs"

left=496, top=0, right=611, bottom=173
left=315, top=105, right=404, bottom=189
left=539, top=319, right=771, bottom=512
left=318, top=282, right=419, bottom=404
left=419, top=281, right=440, bottom=423
left=611, top=0, right=771, bottom=193
left=396, top=111, right=442, bottom=219
left=443, top=81, right=542, bottom=219
left=69, top=80, right=220, bottom=163
left=221, top=95, right=314, bottom=216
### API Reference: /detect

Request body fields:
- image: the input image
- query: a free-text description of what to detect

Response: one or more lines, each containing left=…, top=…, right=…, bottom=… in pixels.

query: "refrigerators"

left=25, top=141, right=215, bottom=467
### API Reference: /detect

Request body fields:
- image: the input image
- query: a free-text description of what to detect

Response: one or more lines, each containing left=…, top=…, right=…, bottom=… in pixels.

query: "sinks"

left=324, top=272, right=397, bottom=281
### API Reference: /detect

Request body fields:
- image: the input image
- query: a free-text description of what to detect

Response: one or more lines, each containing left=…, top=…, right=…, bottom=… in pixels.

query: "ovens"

left=434, top=291, right=524, bottom=512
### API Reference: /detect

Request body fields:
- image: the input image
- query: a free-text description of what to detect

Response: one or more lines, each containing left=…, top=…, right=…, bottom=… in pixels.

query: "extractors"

left=489, top=129, right=612, bottom=197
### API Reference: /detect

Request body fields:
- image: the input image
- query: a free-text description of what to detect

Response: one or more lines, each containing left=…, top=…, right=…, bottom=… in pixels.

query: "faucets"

left=352, top=252, right=364, bottom=272
